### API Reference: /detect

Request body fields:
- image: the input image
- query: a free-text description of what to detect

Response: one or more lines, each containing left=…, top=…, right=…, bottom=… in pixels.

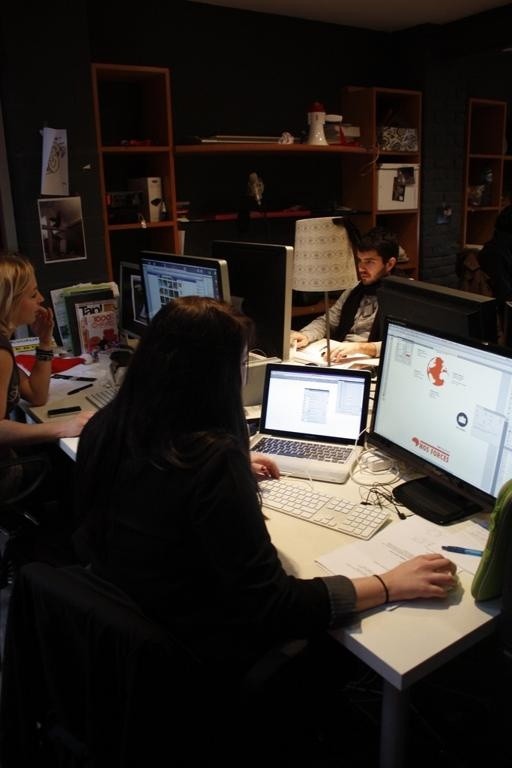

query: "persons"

left=290, top=227, right=404, bottom=362
left=63, top=293, right=458, bottom=766
left=0, top=252, right=95, bottom=442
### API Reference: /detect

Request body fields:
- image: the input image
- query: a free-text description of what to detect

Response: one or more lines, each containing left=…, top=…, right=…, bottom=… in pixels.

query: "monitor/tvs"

left=380, top=274, right=496, bottom=354
left=119, top=262, right=149, bottom=340
left=140, top=250, right=231, bottom=329
left=368, top=318, right=512, bottom=514
left=210, top=239, right=295, bottom=362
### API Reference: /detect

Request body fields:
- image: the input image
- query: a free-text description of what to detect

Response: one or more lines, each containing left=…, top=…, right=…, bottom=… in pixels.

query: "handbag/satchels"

left=394, top=476, right=482, bottom=525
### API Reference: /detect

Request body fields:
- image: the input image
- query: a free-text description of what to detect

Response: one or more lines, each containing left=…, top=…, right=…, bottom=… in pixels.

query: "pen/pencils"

left=441, top=546, right=484, bottom=556
left=67, top=383, right=93, bottom=395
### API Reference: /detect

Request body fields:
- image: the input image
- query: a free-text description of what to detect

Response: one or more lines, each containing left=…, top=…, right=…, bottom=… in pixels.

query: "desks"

left=16, top=351, right=512, bottom=765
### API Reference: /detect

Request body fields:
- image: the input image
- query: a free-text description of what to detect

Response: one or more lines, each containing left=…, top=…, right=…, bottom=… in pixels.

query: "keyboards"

left=86, top=385, right=119, bottom=410
left=257, top=477, right=390, bottom=540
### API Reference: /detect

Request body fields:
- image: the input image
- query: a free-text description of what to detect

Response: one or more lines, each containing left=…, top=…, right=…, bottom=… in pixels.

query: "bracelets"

left=33, top=344, right=54, bottom=362
left=373, top=571, right=394, bottom=607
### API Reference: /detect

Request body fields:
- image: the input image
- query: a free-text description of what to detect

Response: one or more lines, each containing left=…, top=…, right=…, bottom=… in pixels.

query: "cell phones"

left=48, top=406, right=82, bottom=416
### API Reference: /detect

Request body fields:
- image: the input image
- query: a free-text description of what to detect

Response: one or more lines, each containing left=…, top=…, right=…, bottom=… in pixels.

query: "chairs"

left=9, top=561, right=192, bottom=767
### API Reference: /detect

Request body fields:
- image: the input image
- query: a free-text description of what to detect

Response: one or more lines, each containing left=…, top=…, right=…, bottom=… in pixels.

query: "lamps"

left=291, top=216, right=360, bottom=369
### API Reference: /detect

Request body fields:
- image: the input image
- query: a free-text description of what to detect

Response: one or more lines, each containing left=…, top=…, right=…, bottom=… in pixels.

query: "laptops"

left=249, top=363, right=371, bottom=484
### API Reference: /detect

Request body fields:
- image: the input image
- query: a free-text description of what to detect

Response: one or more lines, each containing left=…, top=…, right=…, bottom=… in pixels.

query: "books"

left=45, top=275, right=126, bottom=367
